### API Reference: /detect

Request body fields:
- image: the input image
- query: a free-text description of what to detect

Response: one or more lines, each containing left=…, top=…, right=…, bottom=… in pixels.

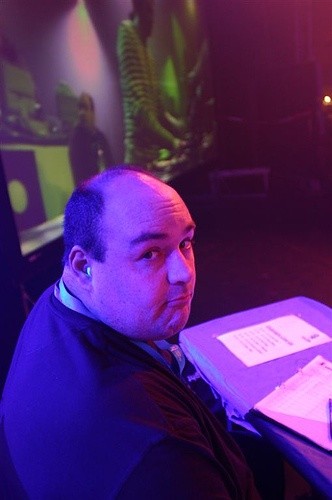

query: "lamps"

left=325, top=96, right=330, bottom=104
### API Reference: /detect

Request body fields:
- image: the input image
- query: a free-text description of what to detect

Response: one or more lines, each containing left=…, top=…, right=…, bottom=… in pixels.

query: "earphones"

left=83, top=264, right=91, bottom=276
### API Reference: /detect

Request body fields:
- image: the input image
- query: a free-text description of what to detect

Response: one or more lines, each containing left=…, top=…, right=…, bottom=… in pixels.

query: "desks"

left=180, top=295, right=332, bottom=500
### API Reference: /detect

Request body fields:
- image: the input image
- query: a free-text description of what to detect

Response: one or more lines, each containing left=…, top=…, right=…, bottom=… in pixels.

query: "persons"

left=115, top=0, right=193, bottom=165
left=0, top=167, right=307, bottom=500
left=66, top=92, right=113, bottom=191
